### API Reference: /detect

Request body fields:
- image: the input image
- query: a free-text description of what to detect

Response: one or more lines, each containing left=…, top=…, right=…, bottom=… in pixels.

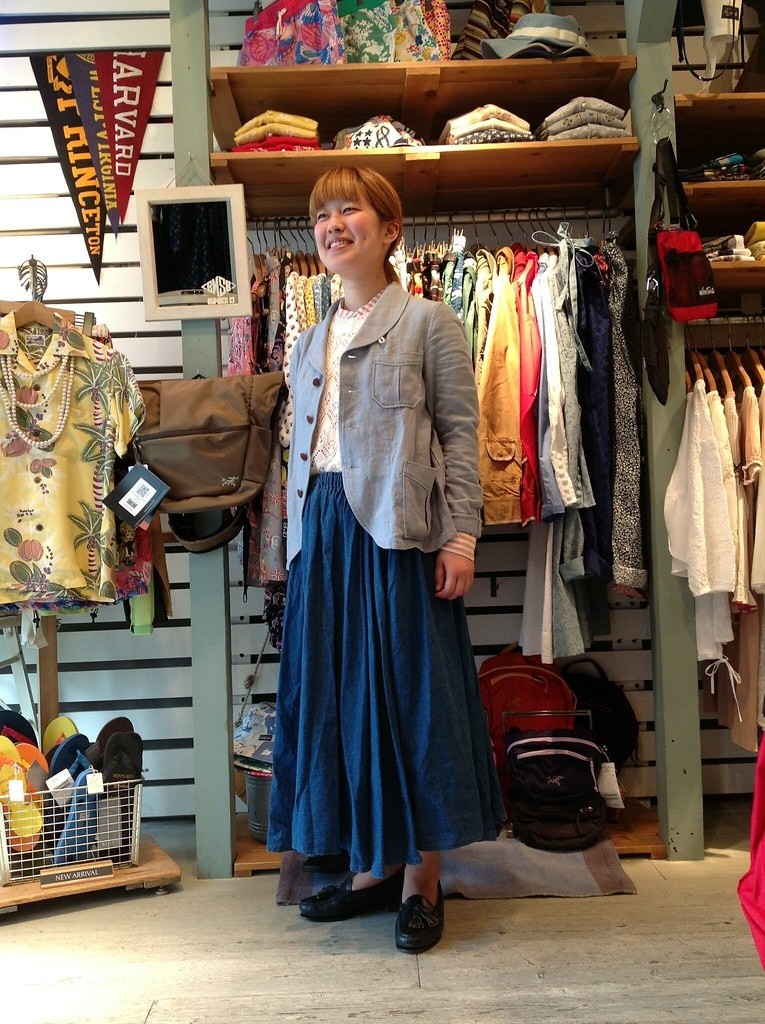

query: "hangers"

left=245, top=207, right=609, bottom=291
left=14, top=253, right=62, bottom=338
left=673, top=313, right=765, bottom=396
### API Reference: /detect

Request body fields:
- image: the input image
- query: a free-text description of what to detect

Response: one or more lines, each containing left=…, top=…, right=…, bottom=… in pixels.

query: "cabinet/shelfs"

left=211, top=56, right=638, bottom=209
left=673, top=91, right=765, bottom=289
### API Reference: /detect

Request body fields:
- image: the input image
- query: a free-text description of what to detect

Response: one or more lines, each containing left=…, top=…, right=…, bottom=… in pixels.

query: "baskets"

left=1, top=774, right=145, bottom=887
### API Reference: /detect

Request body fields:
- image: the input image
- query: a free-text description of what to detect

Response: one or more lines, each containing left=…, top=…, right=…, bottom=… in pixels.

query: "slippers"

left=0, top=708, right=144, bottom=865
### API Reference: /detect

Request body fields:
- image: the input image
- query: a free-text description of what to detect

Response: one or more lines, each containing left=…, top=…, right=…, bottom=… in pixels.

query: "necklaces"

left=0, top=352, right=76, bottom=449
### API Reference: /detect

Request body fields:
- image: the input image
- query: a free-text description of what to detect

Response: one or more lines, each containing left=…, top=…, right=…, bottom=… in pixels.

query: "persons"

left=266, top=164, right=506, bottom=954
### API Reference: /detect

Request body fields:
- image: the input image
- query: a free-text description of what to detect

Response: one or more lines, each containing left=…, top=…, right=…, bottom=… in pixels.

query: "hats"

left=500, top=43, right=595, bottom=61
left=233, top=702, right=276, bottom=776
left=480, top=12, right=589, bottom=59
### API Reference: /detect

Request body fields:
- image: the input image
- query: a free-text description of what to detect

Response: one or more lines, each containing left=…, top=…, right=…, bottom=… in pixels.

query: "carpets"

left=277, top=831, right=638, bottom=904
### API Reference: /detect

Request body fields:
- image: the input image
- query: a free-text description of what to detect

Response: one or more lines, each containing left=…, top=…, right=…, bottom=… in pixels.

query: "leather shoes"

left=299, top=860, right=406, bottom=922
left=395, top=878, right=445, bottom=953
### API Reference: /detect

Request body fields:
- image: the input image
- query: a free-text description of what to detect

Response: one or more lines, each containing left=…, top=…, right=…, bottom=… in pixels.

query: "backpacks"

left=502, top=725, right=621, bottom=853
left=562, top=657, right=639, bottom=768
left=480, top=641, right=577, bottom=814
left=132, top=369, right=284, bottom=553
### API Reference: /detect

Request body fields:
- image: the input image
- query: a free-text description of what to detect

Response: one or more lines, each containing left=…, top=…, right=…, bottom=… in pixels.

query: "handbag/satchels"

left=652, top=225, right=718, bottom=323
left=641, top=287, right=670, bottom=405
left=337, top=0, right=398, bottom=64
left=389, top=1, right=451, bottom=64
left=235, top=0, right=347, bottom=67
